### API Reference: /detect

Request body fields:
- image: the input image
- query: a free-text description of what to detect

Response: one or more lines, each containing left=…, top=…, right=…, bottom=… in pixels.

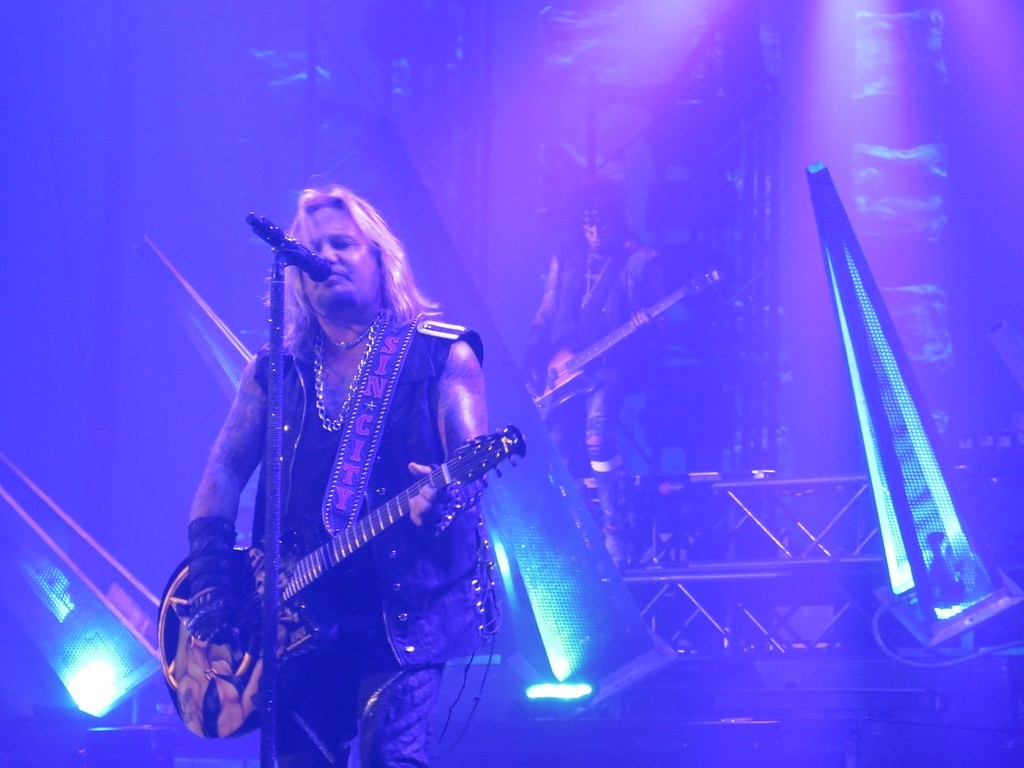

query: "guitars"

left=526, top=266, right=720, bottom=419
left=156, top=424, right=525, bottom=742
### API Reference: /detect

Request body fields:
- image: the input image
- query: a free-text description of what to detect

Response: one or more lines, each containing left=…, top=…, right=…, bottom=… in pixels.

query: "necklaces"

left=311, top=316, right=381, bottom=431
left=578, top=255, right=611, bottom=307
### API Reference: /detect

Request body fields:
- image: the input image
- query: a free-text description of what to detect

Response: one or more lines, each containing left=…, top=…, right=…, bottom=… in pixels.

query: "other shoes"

left=602, top=526, right=626, bottom=568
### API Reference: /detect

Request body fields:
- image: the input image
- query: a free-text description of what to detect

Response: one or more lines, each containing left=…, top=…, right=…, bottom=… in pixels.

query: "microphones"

left=245, top=212, right=332, bottom=283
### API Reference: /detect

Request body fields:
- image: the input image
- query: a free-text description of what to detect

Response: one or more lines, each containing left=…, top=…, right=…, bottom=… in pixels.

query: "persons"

left=187, top=187, right=502, bottom=768
left=523, top=197, right=664, bottom=571
